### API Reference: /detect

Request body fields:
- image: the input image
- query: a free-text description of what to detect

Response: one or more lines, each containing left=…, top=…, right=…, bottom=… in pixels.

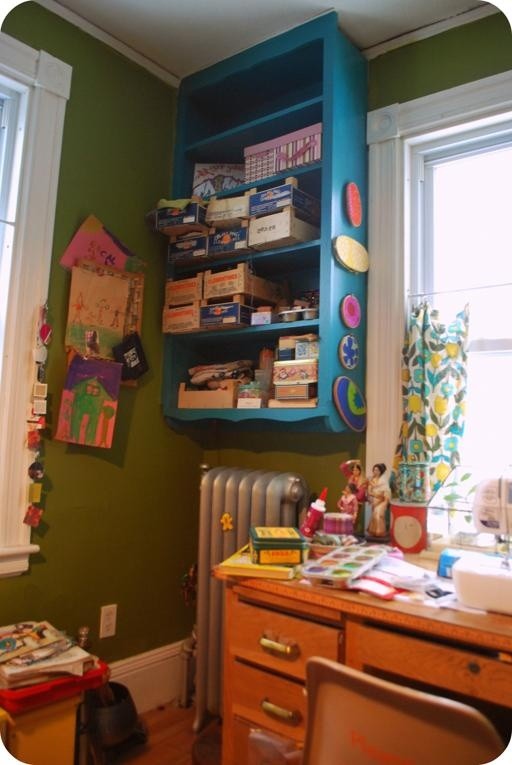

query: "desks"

left=210, top=544, right=510, bottom=764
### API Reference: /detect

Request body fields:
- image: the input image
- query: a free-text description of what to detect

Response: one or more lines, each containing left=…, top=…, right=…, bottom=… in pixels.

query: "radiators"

left=191, top=465, right=308, bottom=733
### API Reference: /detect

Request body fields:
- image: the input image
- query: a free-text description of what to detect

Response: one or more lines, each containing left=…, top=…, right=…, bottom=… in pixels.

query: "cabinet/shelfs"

left=161, top=11, right=369, bottom=436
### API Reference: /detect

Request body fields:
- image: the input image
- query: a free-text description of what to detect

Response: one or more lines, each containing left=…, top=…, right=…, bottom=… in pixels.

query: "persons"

left=337, top=483, right=359, bottom=525
left=340, top=460, right=370, bottom=502
left=367, top=463, right=391, bottom=538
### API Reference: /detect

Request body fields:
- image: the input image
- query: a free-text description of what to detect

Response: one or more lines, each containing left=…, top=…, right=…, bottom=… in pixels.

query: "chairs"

left=299, top=654, right=504, bottom=765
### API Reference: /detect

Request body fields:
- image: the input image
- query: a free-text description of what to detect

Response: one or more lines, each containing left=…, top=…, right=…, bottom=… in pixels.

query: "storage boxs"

left=153, top=121, right=321, bottom=331
